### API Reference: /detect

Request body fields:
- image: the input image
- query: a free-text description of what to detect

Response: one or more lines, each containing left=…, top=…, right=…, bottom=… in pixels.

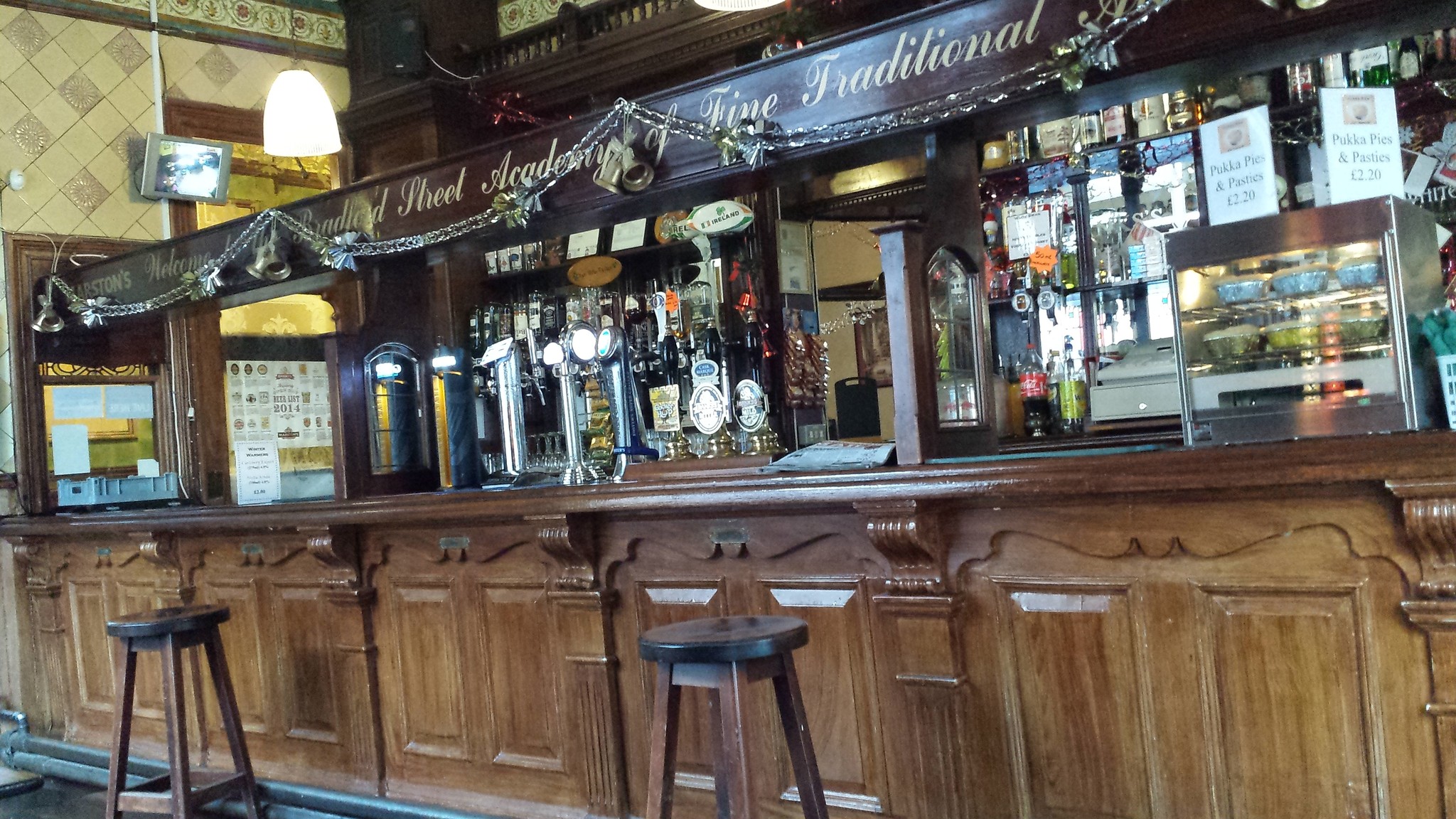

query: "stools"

left=637, top=615, right=829, bottom=819
left=105, top=606, right=263, bottom=819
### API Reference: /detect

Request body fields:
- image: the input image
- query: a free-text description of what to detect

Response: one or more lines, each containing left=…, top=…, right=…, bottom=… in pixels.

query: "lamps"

left=264, top=0, right=342, bottom=157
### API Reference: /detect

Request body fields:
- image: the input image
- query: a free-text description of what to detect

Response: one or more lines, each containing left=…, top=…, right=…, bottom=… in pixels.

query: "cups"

left=1133, top=95, right=1167, bottom=137
left=982, top=140, right=1010, bottom=170
left=1038, top=114, right=1079, bottom=159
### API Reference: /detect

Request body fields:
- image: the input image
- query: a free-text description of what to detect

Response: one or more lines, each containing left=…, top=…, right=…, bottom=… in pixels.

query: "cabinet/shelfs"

left=1163, top=189, right=1443, bottom=447
left=976, top=78, right=1456, bottom=450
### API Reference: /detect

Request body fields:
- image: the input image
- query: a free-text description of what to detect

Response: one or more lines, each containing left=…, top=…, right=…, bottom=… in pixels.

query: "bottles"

left=1007, top=68, right=1272, bottom=167
left=467, top=266, right=690, bottom=351
left=1286, top=29, right=1456, bottom=102
left=999, top=339, right=1087, bottom=438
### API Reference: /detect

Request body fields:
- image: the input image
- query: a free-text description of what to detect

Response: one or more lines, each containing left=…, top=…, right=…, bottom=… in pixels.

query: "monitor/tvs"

left=140, top=133, right=233, bottom=206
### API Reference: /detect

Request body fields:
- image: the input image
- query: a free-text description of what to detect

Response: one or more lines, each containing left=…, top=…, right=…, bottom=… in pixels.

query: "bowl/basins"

left=1335, top=262, right=1381, bottom=290
left=1203, top=332, right=1259, bottom=360
left=1265, top=325, right=1316, bottom=346
left=1213, top=280, right=1271, bottom=303
left=1272, top=270, right=1328, bottom=298
left=1335, top=315, right=1390, bottom=340
